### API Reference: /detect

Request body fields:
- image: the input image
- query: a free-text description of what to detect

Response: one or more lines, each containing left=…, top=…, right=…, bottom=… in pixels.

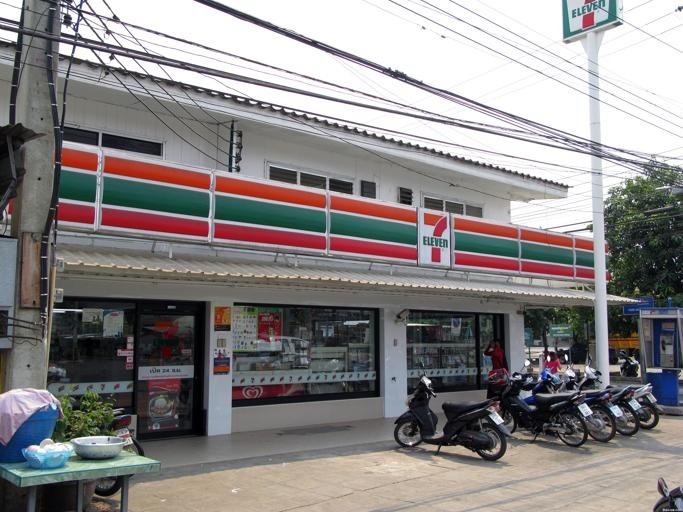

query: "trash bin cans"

left=646, top=369, right=681, bottom=407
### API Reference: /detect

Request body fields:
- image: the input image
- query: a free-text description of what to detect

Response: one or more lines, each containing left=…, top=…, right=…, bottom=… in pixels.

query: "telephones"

left=660, top=333, right=674, bottom=368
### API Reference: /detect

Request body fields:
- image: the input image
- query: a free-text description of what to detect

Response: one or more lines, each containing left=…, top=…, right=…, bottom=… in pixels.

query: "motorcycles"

left=653, top=476, right=683, bottom=512
left=94, top=406, right=146, bottom=497
left=393, top=346, right=665, bottom=462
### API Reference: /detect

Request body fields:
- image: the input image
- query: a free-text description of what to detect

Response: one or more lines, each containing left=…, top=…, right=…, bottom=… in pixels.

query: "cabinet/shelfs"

left=306, top=343, right=476, bottom=394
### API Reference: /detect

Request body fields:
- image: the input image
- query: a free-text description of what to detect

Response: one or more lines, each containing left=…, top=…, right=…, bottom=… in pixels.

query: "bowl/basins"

left=69, top=436, right=128, bottom=460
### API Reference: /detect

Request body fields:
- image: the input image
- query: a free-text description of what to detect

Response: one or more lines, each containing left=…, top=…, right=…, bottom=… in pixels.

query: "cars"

left=570, top=340, right=618, bottom=364
left=266, top=336, right=310, bottom=349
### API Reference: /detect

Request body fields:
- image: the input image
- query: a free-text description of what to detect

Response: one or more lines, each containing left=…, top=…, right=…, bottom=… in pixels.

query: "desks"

left=0, top=449, right=162, bottom=512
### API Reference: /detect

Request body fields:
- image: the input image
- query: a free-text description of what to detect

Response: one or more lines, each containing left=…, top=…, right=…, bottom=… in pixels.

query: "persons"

left=484, top=338, right=508, bottom=399
left=544, top=351, right=562, bottom=380
